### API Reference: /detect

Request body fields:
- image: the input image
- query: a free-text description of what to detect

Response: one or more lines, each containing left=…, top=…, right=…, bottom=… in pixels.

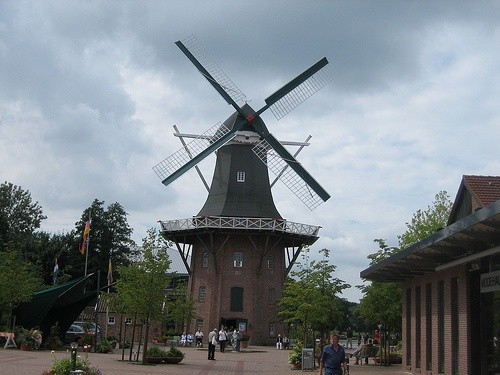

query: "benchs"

left=276, top=337, right=300, bottom=349
left=167, top=336, right=193, bottom=346
left=357, top=345, right=378, bottom=366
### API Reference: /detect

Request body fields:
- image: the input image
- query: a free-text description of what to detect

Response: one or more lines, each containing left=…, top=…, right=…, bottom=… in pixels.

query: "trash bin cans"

left=301, top=348, right=314, bottom=372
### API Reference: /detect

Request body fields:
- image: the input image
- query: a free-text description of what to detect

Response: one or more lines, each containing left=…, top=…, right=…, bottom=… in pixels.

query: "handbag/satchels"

left=212, top=335, right=218, bottom=345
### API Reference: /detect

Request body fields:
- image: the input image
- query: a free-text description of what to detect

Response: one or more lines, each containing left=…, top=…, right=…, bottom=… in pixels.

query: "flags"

left=52, top=259, right=58, bottom=281
left=78, top=218, right=90, bottom=254
left=106, top=261, right=114, bottom=284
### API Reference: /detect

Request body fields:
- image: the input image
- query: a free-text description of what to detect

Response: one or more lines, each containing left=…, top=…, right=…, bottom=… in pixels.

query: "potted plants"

left=145, top=344, right=164, bottom=364
left=82, top=334, right=93, bottom=353
left=106, top=335, right=118, bottom=349
left=164, top=345, right=184, bottom=364
left=238, top=333, right=251, bottom=349
left=18, top=326, right=36, bottom=352
left=288, top=340, right=304, bottom=370
left=96, top=339, right=110, bottom=353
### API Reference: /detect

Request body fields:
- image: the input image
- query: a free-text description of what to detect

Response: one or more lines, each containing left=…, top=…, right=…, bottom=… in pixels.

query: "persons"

left=276, top=333, right=283, bottom=349
left=319, top=334, right=350, bottom=375
left=49, top=325, right=58, bottom=356
left=207, top=328, right=218, bottom=361
left=345, top=328, right=399, bottom=365
left=179, top=325, right=244, bottom=353
left=30, top=325, right=42, bottom=349
left=282, top=334, right=290, bottom=349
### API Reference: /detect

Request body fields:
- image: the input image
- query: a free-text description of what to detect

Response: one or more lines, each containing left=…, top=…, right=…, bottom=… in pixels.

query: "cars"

left=64, top=321, right=100, bottom=347
